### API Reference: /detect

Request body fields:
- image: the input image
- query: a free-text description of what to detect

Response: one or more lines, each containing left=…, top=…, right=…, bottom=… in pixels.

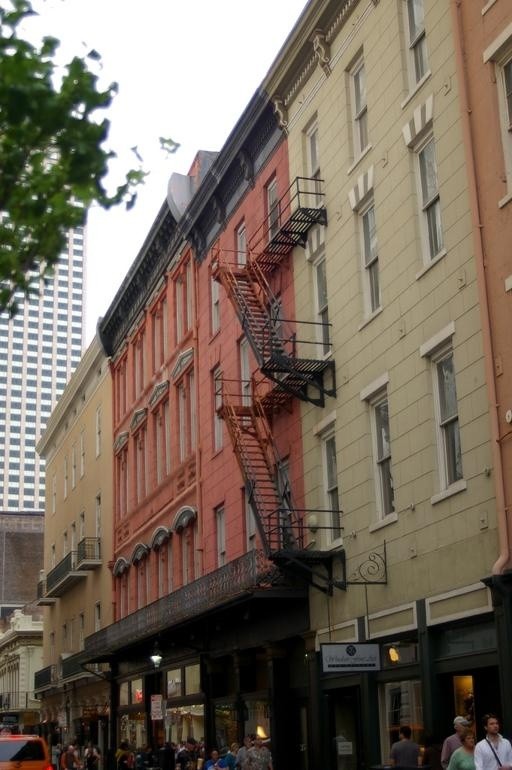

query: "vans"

left=0, top=735, right=52, bottom=770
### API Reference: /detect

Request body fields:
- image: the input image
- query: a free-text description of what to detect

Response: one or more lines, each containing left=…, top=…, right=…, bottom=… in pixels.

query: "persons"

left=473, top=716, right=512, bottom=770
left=389, top=726, right=420, bottom=770
left=446, top=728, right=476, bottom=770
left=53, top=735, right=273, bottom=770
left=440, top=716, right=470, bottom=769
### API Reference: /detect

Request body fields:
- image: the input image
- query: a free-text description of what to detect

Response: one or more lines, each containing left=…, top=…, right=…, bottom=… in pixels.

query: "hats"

left=453, top=716, right=470, bottom=726
left=187, top=736, right=197, bottom=745
left=119, top=743, right=130, bottom=752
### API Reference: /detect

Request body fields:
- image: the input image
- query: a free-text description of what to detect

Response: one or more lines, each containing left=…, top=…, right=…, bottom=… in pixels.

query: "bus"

left=0, top=711, right=40, bottom=728
